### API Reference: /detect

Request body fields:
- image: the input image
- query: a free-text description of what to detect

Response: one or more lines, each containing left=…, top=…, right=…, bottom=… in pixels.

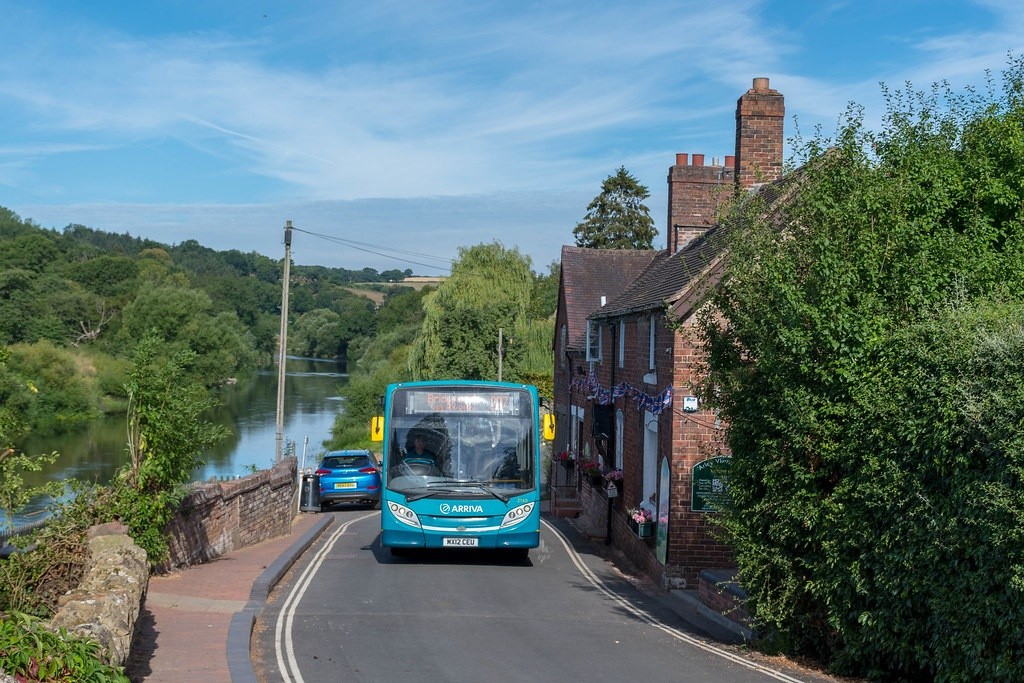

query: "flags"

left=567, top=370, right=673, bottom=415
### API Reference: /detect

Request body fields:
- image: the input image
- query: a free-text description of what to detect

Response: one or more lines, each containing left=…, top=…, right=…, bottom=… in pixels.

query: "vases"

left=582, top=469, right=602, bottom=485
left=626, top=514, right=652, bottom=537
left=560, top=459, right=575, bottom=468
left=601, top=476, right=624, bottom=491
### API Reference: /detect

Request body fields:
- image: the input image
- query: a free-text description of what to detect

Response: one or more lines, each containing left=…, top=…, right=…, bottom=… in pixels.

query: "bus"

left=372, top=380, right=556, bottom=560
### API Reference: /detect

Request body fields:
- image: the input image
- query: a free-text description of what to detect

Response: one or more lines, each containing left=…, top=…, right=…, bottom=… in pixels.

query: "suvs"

left=315, top=449, right=382, bottom=510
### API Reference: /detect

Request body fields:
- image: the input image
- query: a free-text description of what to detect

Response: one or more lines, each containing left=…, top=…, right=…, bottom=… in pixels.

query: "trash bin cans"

left=299, top=474, right=321, bottom=513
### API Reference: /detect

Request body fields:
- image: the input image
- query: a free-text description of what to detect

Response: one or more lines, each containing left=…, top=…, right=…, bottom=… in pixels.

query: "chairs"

left=327, top=457, right=367, bottom=467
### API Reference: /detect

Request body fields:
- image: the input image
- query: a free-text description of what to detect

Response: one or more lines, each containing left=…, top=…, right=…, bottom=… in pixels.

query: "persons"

left=403, top=437, right=434, bottom=463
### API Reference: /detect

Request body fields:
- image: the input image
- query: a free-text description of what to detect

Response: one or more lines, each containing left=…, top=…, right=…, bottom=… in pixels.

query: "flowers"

left=560, top=450, right=576, bottom=461
left=582, top=459, right=601, bottom=476
left=604, top=469, right=623, bottom=481
left=625, top=506, right=652, bottom=523
